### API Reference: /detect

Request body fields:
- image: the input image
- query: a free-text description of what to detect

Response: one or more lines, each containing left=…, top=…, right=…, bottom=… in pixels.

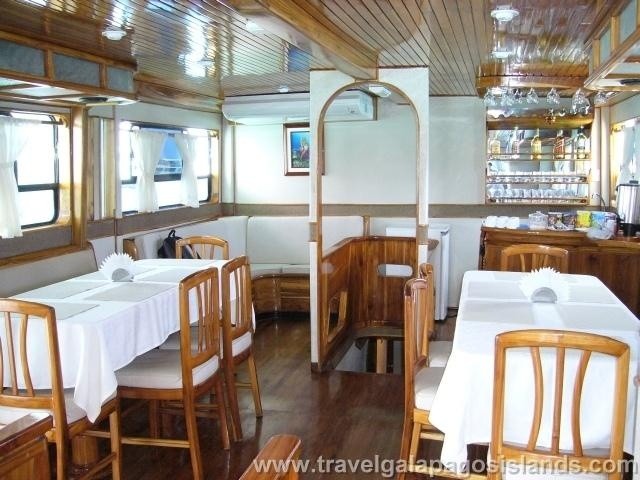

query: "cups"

left=483, top=215, right=520, bottom=229
left=489, top=171, right=588, bottom=182
left=488, top=188, right=576, bottom=203
left=547, top=210, right=617, bottom=229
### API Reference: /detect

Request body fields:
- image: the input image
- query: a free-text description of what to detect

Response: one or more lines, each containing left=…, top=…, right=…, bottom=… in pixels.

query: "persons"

left=298, top=137, right=309, bottom=161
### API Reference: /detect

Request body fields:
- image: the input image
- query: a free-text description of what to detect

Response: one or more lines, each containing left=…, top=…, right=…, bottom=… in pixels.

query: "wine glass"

left=482, top=86, right=607, bottom=117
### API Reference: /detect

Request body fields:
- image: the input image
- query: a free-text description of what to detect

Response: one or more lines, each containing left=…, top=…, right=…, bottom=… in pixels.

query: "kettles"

left=615, top=181, right=639, bottom=236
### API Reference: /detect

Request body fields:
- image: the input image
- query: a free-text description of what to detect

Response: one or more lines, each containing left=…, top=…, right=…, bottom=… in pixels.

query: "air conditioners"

left=222, top=92, right=377, bottom=124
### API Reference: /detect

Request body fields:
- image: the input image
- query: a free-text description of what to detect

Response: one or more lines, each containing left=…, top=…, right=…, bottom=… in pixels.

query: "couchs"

left=0, top=243, right=98, bottom=297
left=117, top=214, right=250, bottom=270
left=249, top=214, right=370, bottom=316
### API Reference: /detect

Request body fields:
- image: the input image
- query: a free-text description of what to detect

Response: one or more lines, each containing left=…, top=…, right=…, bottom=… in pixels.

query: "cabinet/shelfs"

left=487, top=94, right=593, bottom=206
left=480, top=226, right=640, bottom=317
left=582, top=0, right=640, bottom=91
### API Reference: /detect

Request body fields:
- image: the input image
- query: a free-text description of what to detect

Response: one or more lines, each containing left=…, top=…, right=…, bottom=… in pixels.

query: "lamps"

left=492, top=9, right=520, bottom=22
left=102, top=31, right=127, bottom=42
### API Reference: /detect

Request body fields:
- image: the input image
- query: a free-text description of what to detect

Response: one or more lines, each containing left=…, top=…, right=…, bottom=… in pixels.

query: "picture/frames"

left=283, top=123, right=325, bottom=176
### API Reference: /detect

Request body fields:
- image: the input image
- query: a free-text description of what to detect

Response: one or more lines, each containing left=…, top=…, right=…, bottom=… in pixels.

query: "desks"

left=0, top=406, right=53, bottom=479
left=0, top=258, right=249, bottom=480
left=441, top=271, right=640, bottom=480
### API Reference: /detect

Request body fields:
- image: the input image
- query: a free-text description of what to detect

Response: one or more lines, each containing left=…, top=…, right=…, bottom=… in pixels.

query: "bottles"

left=510, top=126, right=520, bottom=158
left=528, top=210, right=548, bottom=231
left=490, top=130, right=500, bottom=159
left=531, top=127, right=541, bottom=159
left=553, top=129, right=565, bottom=159
left=576, top=126, right=586, bottom=159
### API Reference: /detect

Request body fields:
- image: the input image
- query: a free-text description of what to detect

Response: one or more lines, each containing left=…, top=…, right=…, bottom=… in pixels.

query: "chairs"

left=114, top=267, right=232, bottom=480
left=501, top=242, right=570, bottom=273
left=176, top=236, right=229, bottom=259
left=1, top=298, right=123, bottom=480
left=486, top=329, right=630, bottom=479
left=396, top=277, right=490, bottom=480
left=160, top=255, right=263, bottom=441
left=419, top=263, right=452, bottom=368
